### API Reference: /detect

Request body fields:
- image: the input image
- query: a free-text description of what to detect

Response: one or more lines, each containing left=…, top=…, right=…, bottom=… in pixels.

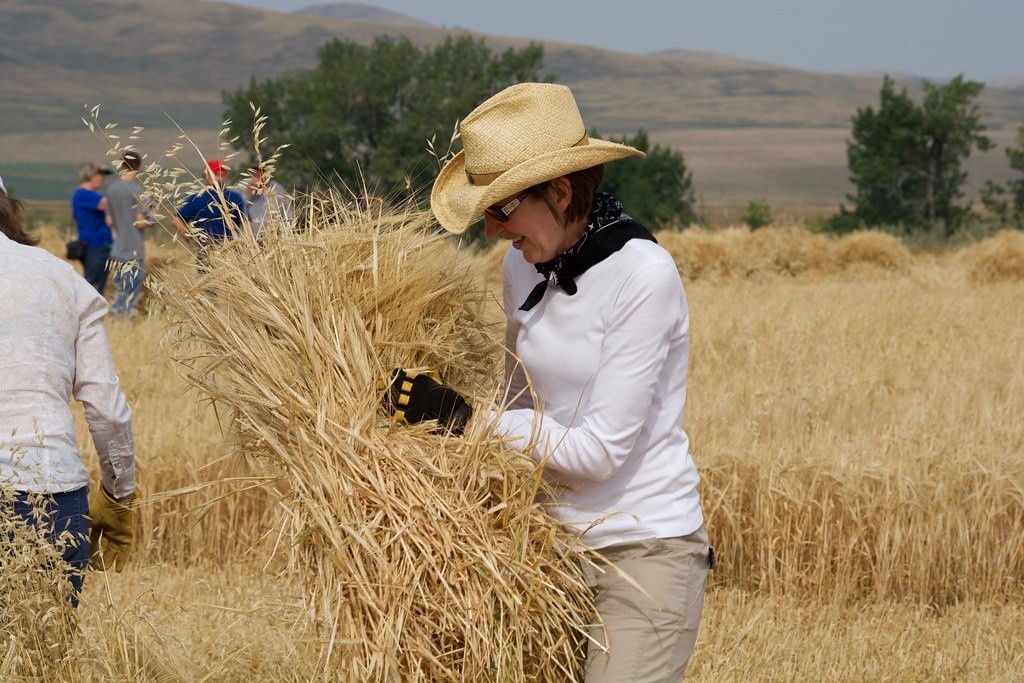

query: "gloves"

left=376, top=367, right=473, bottom=437
left=88, top=479, right=137, bottom=573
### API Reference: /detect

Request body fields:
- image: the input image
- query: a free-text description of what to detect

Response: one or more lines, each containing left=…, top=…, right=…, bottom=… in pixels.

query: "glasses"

left=486, top=186, right=539, bottom=222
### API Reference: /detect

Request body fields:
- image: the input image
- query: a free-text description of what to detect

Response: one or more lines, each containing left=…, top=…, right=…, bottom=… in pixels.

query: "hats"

left=0, top=176, right=7, bottom=197
left=204, top=160, right=227, bottom=176
left=250, top=160, right=272, bottom=172
left=121, top=151, right=141, bottom=171
left=430, top=82, right=647, bottom=236
left=79, top=161, right=113, bottom=181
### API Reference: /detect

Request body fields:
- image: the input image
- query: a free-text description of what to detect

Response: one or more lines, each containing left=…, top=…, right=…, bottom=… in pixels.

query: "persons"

left=232, top=150, right=292, bottom=241
left=105, top=151, right=155, bottom=318
left=0, top=174, right=136, bottom=683
left=371, top=82, right=710, bottom=683
left=171, top=160, right=254, bottom=298
left=71, top=162, right=115, bottom=296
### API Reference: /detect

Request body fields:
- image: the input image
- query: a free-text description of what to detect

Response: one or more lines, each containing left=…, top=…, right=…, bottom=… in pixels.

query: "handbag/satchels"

left=66, top=240, right=87, bottom=259
